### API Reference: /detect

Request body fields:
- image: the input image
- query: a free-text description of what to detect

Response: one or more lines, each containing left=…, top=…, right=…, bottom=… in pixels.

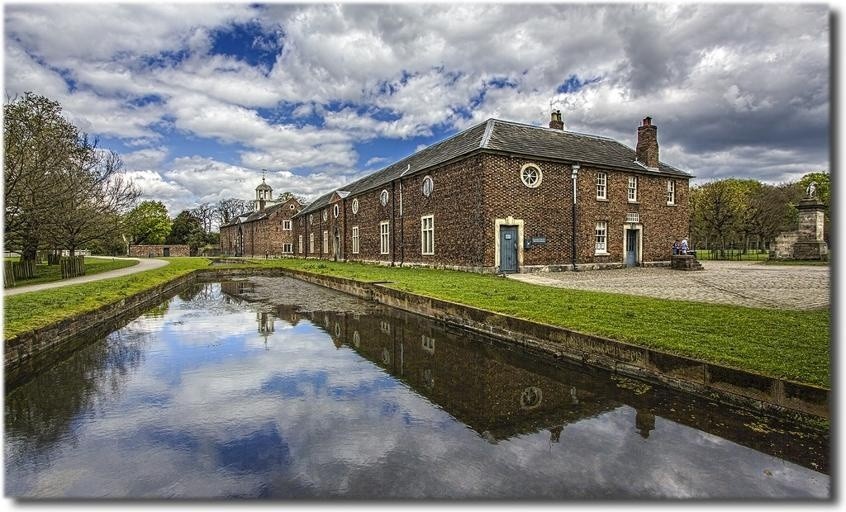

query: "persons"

left=681, top=237, right=689, bottom=255
left=672, top=240, right=679, bottom=255
